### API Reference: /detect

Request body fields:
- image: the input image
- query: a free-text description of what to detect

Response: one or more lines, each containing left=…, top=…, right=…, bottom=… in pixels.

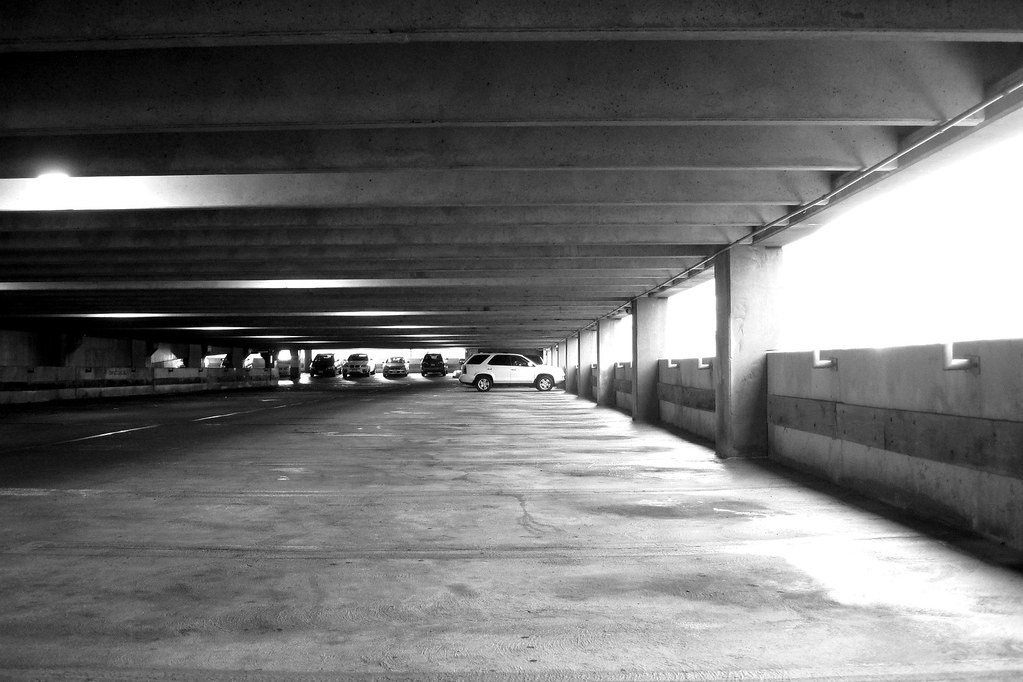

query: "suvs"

left=458, top=352, right=567, bottom=393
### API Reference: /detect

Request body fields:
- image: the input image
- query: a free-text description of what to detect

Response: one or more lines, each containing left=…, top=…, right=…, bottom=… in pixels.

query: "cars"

left=382, top=356, right=410, bottom=378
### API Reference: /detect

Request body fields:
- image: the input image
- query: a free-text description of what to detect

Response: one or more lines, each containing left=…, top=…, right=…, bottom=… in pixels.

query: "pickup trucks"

left=341, top=353, right=376, bottom=377
left=309, top=353, right=343, bottom=377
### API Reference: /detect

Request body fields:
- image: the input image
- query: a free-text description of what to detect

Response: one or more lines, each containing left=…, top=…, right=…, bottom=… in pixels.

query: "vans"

left=420, top=352, right=447, bottom=376
